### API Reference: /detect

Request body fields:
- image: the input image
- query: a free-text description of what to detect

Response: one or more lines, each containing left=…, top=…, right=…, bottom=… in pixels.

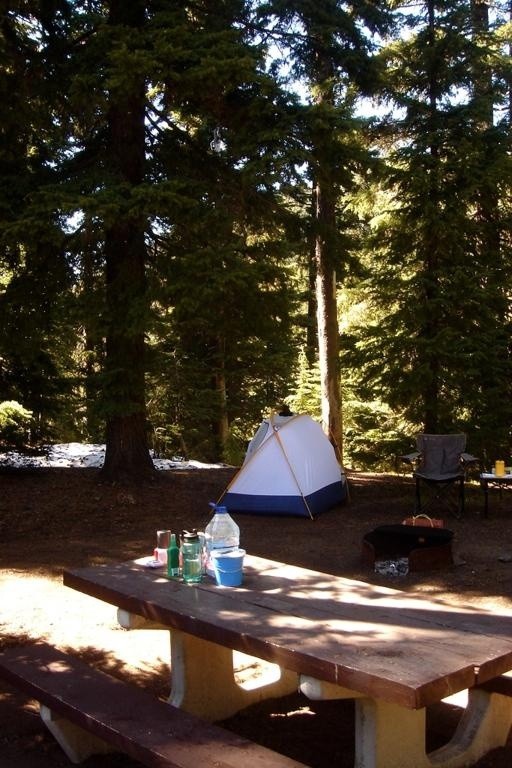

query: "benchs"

left=1, top=644, right=310, bottom=768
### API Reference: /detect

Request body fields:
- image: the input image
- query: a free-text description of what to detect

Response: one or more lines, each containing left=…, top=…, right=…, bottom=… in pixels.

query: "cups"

left=181, top=532, right=202, bottom=583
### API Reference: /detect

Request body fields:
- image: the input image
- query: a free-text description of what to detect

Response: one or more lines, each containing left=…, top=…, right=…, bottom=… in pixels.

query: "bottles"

left=496, top=461, right=505, bottom=476
left=154, top=530, right=179, bottom=577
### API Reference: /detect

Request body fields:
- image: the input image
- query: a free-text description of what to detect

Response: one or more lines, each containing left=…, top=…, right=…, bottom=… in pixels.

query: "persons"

left=279, top=404, right=292, bottom=416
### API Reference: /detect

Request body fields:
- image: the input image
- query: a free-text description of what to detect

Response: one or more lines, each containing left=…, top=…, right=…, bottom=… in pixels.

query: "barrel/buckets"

left=205, top=501, right=240, bottom=578
left=210, top=548, right=246, bottom=586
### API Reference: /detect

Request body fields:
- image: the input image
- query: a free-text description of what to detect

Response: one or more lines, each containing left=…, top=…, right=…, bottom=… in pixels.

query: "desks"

left=480, top=472, right=512, bottom=518
left=63, top=553, right=512, bottom=768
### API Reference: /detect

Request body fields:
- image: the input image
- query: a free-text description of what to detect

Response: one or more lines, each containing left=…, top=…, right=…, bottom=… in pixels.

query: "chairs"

left=400, top=434, right=479, bottom=517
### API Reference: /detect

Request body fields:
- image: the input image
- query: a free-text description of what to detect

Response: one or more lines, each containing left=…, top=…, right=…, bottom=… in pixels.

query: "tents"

left=209, top=412, right=353, bottom=519
